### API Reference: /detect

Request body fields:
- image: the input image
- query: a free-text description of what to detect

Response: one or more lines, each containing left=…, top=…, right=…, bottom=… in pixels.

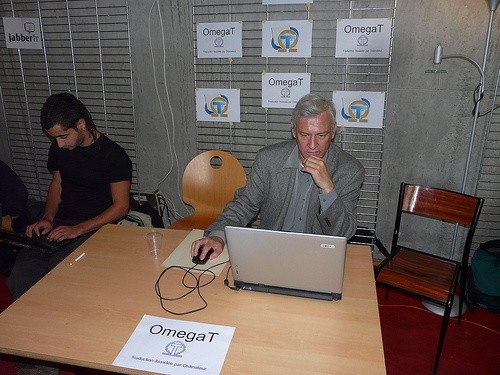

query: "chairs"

left=371, top=182, right=484, bottom=375
left=168, top=150, right=247, bottom=231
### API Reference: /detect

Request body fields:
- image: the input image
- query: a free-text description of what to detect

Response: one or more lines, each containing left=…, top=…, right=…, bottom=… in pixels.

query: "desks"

left=0, top=224, right=387, bottom=375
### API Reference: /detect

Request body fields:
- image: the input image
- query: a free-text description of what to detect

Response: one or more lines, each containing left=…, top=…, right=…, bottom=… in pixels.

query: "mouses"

left=192, top=246, right=214, bottom=264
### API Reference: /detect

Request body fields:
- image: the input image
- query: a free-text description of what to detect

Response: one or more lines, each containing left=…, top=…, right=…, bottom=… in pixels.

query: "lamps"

left=421, top=0, right=500, bottom=317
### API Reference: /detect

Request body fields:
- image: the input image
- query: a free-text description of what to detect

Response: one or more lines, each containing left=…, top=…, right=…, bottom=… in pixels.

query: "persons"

left=0, top=92, right=133, bottom=299
left=190, top=93, right=366, bottom=261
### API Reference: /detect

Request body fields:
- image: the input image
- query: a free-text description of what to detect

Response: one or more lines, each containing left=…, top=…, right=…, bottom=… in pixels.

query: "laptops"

left=225, top=226, right=347, bottom=301
left=0, top=229, right=82, bottom=254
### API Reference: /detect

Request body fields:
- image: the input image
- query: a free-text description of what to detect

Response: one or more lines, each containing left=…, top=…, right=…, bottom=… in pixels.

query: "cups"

left=145, top=231, right=163, bottom=256
